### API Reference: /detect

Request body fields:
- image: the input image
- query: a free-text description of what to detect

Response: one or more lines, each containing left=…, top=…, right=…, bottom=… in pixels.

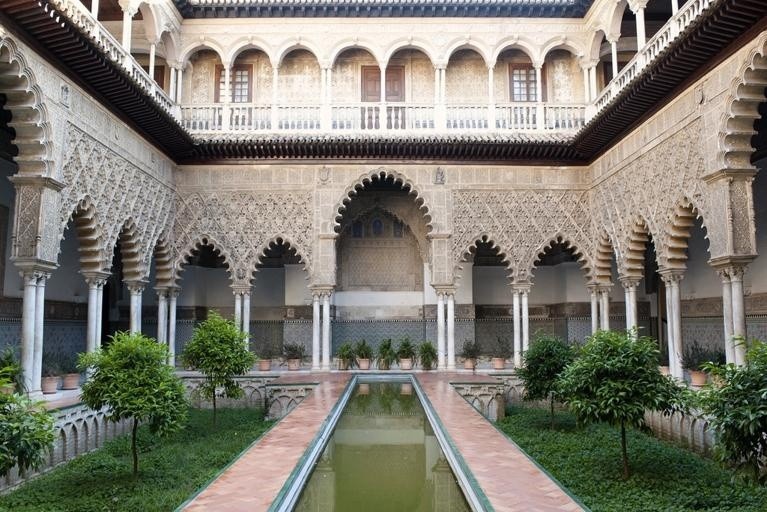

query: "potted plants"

left=463, top=339, right=481, bottom=369
left=354, top=338, right=372, bottom=370
left=397, top=383, right=416, bottom=415
left=355, top=384, right=372, bottom=414
left=396, top=338, right=418, bottom=370
left=335, top=338, right=354, bottom=369
left=485, top=335, right=513, bottom=369
left=60, top=354, right=80, bottom=389
left=280, top=341, right=305, bottom=369
left=377, top=383, right=397, bottom=415
left=2, top=344, right=31, bottom=397
left=41, top=353, right=59, bottom=394
left=258, top=349, right=272, bottom=370
left=375, top=338, right=399, bottom=370
left=678, top=339, right=711, bottom=385
